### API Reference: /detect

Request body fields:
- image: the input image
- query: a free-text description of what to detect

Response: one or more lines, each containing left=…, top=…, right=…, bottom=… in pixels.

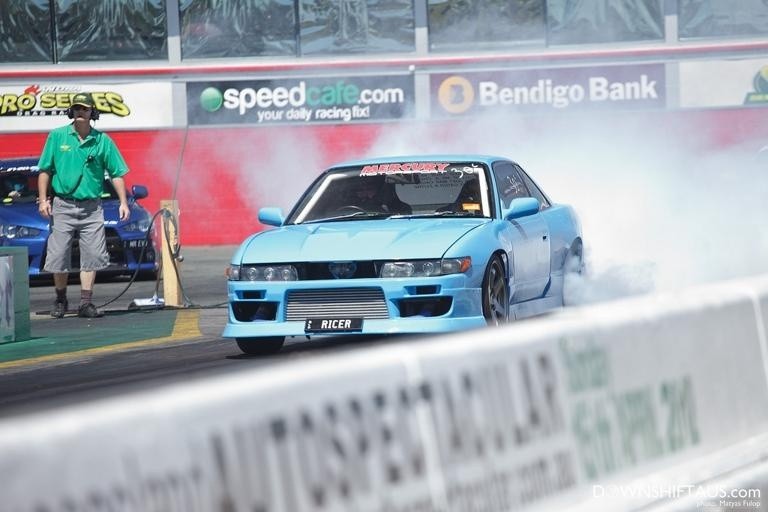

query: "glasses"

left=73, top=107, right=92, bottom=111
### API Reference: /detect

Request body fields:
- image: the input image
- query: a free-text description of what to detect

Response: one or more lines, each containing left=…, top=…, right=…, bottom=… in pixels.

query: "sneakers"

left=50, top=299, right=67, bottom=318
left=79, top=303, right=105, bottom=318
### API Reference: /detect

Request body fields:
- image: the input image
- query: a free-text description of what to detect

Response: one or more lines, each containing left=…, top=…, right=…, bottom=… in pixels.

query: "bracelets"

left=36, top=197, right=51, bottom=205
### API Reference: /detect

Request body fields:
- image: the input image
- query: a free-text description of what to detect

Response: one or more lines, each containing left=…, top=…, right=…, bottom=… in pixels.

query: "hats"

left=70, top=93, right=93, bottom=109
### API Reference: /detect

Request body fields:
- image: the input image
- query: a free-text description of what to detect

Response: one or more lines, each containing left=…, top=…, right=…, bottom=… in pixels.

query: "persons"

left=36, top=93, right=131, bottom=319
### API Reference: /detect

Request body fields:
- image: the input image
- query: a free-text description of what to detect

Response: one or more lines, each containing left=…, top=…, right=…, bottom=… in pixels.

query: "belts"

left=60, top=197, right=88, bottom=202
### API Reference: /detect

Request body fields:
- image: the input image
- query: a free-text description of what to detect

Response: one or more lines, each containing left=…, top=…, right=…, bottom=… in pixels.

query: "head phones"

left=66, top=93, right=101, bottom=120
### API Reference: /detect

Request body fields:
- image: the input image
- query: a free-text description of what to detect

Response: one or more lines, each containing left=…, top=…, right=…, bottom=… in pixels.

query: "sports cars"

left=221, top=154, right=589, bottom=357
left=0, top=157, right=155, bottom=284
